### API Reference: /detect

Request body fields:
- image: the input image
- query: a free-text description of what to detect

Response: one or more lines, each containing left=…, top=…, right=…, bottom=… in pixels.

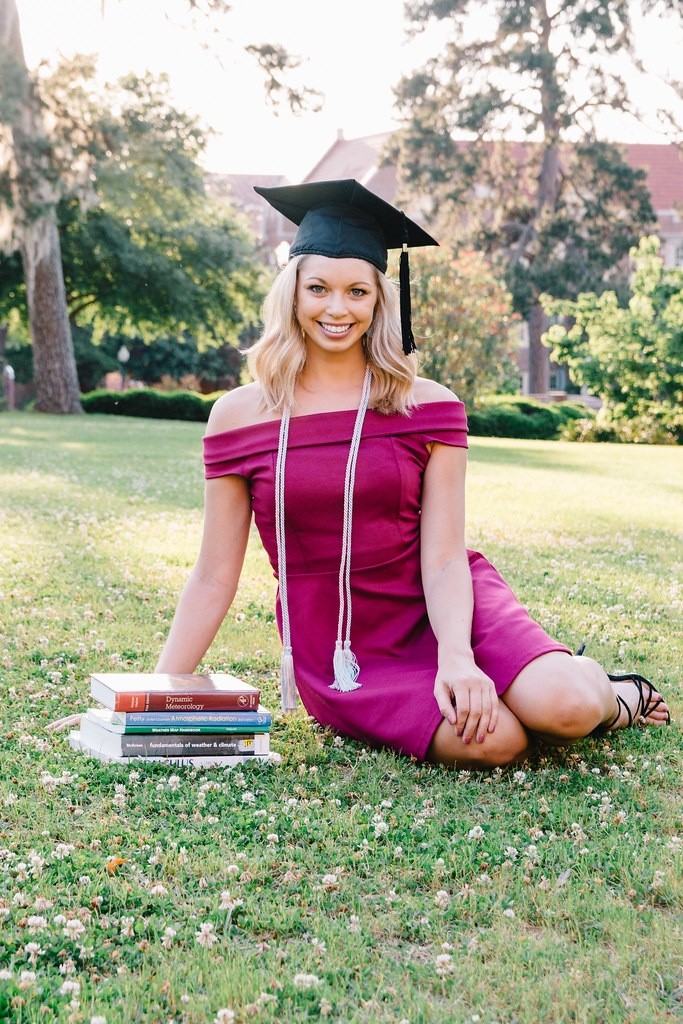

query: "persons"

left=41, top=180, right=672, bottom=767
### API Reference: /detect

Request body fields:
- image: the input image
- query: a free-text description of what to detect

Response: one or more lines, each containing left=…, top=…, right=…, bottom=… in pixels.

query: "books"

left=68, top=671, right=282, bottom=769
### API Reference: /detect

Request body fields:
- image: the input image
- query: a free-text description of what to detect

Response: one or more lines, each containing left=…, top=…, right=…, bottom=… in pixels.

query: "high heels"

left=574, top=640, right=673, bottom=730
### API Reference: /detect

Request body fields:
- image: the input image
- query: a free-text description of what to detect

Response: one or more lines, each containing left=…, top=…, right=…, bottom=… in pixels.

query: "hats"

left=252, top=178, right=440, bottom=276
